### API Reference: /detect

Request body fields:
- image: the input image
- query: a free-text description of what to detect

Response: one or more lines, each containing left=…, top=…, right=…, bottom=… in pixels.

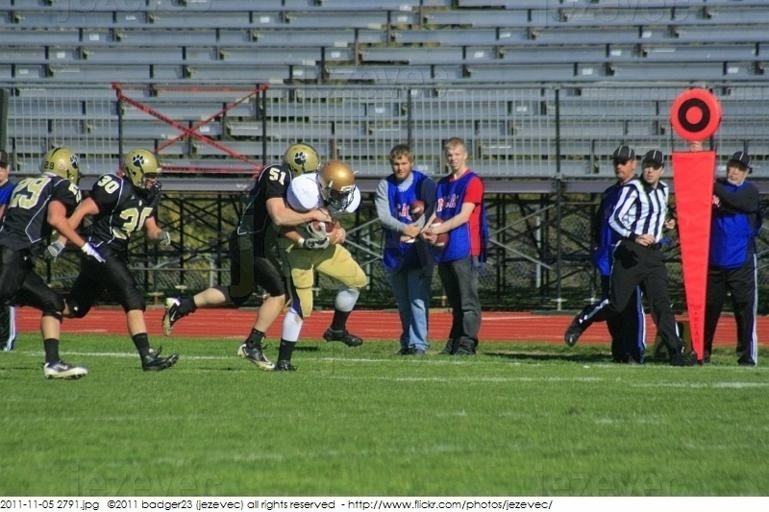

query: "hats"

left=609, top=146, right=635, bottom=159
left=729, top=151, right=751, bottom=168
left=643, top=150, right=665, bottom=168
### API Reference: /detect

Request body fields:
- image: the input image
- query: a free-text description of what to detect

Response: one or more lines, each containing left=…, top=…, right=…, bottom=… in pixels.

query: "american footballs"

left=410, top=199, right=426, bottom=223
left=430, top=218, right=449, bottom=249
left=309, top=218, right=335, bottom=233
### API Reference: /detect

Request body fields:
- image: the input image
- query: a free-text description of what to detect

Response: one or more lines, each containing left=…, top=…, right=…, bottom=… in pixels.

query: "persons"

left=0, top=147, right=179, bottom=380
left=704, top=151, right=762, bottom=367
left=592, top=145, right=647, bottom=364
left=162, top=143, right=333, bottom=371
left=420, top=138, right=489, bottom=356
left=375, top=144, right=437, bottom=355
left=564, top=149, right=698, bottom=366
left=275, top=163, right=368, bottom=371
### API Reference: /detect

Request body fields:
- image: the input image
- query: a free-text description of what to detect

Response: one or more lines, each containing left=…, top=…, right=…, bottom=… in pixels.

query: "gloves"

left=305, top=221, right=327, bottom=238
left=156, top=230, right=171, bottom=247
left=81, top=241, right=106, bottom=263
left=42, top=240, right=65, bottom=263
left=302, top=236, right=331, bottom=250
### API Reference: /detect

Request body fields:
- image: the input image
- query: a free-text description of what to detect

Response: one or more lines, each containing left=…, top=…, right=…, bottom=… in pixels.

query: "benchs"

left=0, top=0, right=769, bottom=180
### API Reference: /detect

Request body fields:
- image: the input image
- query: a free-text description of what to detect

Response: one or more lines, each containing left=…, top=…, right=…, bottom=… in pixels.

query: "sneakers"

left=564, top=318, right=586, bottom=347
left=43, top=297, right=363, bottom=380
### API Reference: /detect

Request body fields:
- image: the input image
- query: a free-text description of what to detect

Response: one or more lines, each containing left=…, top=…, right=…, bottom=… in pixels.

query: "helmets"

left=38, top=143, right=355, bottom=211
